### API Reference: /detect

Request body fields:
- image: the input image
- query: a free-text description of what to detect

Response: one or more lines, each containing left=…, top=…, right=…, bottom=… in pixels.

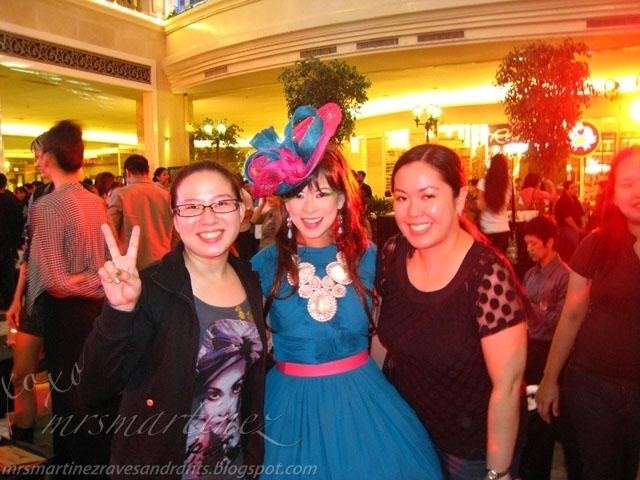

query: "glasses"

left=173, top=200, right=239, bottom=217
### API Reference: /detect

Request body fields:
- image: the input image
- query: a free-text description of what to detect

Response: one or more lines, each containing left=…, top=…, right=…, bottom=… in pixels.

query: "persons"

left=0, top=100, right=640, bottom=480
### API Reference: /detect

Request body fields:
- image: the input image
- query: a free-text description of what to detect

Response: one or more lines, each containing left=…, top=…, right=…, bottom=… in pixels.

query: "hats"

left=243, top=101, right=343, bottom=199
